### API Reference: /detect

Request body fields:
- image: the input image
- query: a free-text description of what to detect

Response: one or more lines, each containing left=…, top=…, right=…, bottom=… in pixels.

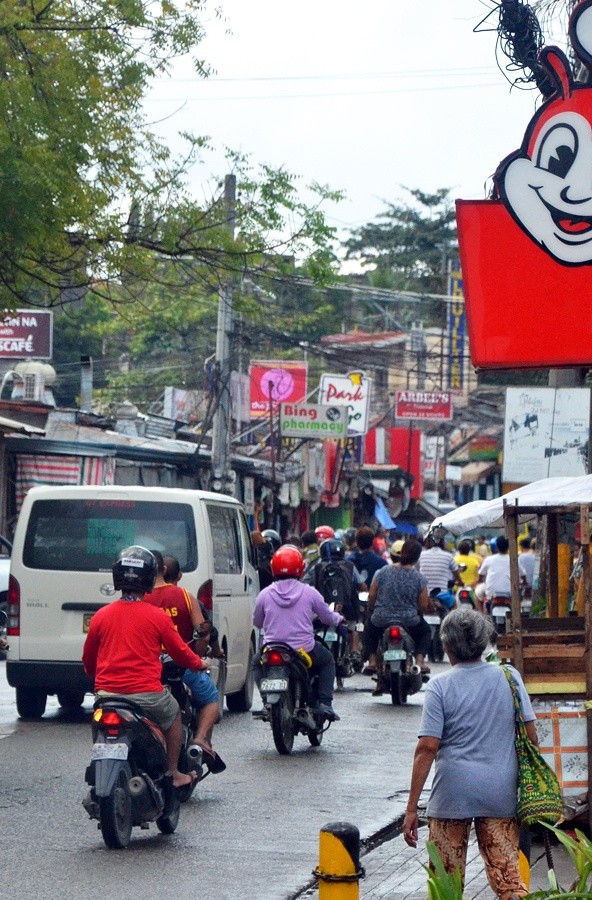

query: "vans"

left=6, top=484, right=260, bottom=719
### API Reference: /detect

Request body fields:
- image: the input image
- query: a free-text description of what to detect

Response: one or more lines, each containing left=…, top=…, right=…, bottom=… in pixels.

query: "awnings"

left=453, top=462, right=496, bottom=485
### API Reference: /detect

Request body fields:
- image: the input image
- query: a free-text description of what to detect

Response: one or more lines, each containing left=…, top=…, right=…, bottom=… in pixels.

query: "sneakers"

left=320, top=703, right=340, bottom=721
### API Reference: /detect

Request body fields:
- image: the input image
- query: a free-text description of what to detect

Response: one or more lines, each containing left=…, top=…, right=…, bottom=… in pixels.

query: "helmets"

left=315, top=525, right=335, bottom=541
left=262, top=529, right=282, bottom=548
left=112, top=546, right=156, bottom=594
left=270, top=545, right=305, bottom=578
left=319, top=540, right=346, bottom=559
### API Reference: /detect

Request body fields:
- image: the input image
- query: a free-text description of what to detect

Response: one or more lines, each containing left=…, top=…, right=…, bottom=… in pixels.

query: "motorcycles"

left=365, top=619, right=429, bottom=706
left=155, top=651, right=214, bottom=803
left=488, top=592, right=514, bottom=634
left=80, top=620, right=213, bottom=849
left=313, top=624, right=361, bottom=688
left=455, top=586, right=474, bottom=608
left=421, top=596, right=450, bottom=663
left=252, top=613, right=331, bottom=755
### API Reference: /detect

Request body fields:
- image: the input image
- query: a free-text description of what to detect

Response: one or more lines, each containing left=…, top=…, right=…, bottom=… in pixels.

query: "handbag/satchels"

left=503, top=666, right=564, bottom=827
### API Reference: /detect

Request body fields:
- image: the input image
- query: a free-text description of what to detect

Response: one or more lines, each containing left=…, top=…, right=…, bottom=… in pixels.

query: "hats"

left=391, top=541, right=405, bottom=557
left=484, top=537, right=498, bottom=551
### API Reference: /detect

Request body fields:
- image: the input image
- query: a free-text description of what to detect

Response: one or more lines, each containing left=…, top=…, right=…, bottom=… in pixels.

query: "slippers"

left=421, top=667, right=431, bottom=673
left=175, top=764, right=201, bottom=801
left=363, top=667, right=376, bottom=675
left=192, top=743, right=227, bottom=773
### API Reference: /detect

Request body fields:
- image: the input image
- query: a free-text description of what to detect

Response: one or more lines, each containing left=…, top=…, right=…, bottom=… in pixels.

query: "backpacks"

left=315, top=561, right=358, bottom=621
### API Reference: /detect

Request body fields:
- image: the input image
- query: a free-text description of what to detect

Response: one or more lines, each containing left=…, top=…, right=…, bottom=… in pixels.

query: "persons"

left=142, top=551, right=221, bottom=762
left=81, top=546, right=210, bottom=787
left=251, top=501, right=581, bottom=673
left=252, top=546, right=346, bottom=720
left=402, top=609, right=536, bottom=900
